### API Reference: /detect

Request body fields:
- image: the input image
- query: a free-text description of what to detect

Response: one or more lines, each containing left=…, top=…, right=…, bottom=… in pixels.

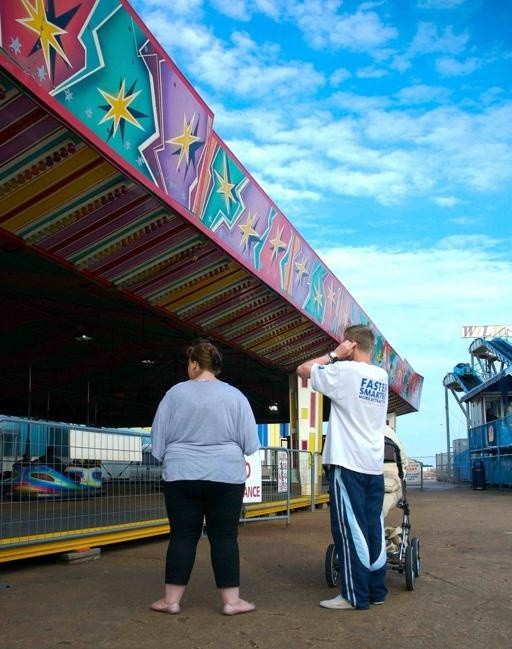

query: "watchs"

left=328, top=351, right=338, bottom=364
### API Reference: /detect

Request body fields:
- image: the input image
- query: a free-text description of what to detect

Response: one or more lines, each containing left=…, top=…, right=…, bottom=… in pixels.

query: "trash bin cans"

left=472, top=461, right=486, bottom=491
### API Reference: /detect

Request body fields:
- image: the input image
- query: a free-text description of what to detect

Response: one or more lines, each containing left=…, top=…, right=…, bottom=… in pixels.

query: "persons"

left=296, top=324, right=388, bottom=610
left=150, top=339, right=258, bottom=615
left=38, top=446, right=67, bottom=476
left=487, top=408, right=499, bottom=422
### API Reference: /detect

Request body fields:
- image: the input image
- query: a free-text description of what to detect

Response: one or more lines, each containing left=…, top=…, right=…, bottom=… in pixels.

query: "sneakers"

left=319, top=594, right=357, bottom=610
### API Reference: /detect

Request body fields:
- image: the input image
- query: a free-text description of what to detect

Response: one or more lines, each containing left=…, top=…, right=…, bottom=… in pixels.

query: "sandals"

left=223, top=598, right=255, bottom=616
left=149, top=597, right=181, bottom=614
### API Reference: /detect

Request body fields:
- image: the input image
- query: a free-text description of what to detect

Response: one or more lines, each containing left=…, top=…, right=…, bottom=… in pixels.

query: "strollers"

left=325, top=425, right=420, bottom=592
